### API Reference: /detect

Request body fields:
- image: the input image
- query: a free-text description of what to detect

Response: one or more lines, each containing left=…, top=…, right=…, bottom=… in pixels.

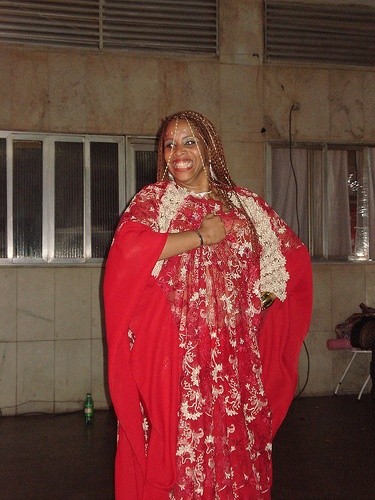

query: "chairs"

left=334, top=349, right=373, bottom=400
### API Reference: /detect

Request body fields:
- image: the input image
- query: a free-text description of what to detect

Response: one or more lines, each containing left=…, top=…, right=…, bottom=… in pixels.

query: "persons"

left=102, top=111, right=313, bottom=500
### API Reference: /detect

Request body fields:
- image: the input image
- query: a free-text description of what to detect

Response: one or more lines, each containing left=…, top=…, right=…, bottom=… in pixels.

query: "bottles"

left=84, top=393, right=94, bottom=424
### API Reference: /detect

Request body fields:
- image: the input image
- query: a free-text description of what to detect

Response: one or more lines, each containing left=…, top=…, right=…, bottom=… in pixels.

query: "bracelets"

left=194, top=230, right=203, bottom=245
